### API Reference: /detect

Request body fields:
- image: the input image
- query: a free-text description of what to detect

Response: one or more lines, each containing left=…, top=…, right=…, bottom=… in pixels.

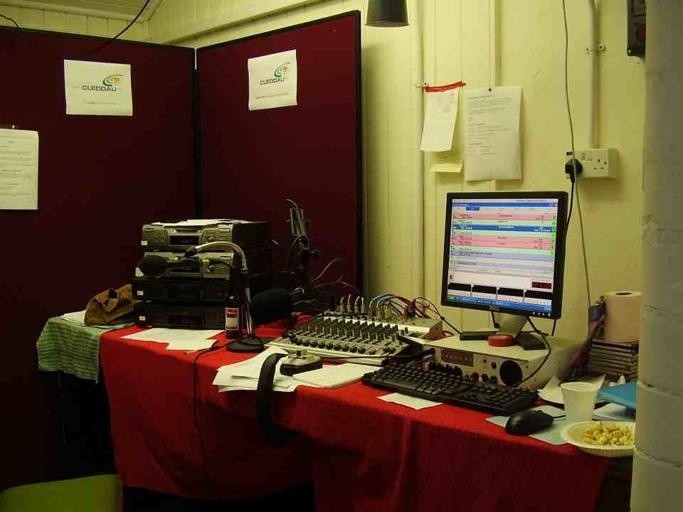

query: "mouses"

left=505, top=409, right=554, bottom=435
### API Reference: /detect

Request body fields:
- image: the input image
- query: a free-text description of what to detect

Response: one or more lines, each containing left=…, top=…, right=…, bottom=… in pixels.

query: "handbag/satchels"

left=84, top=284, right=134, bottom=326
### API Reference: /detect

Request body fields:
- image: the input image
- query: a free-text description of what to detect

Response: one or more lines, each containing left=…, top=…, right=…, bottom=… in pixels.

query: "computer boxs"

left=422, top=328, right=588, bottom=389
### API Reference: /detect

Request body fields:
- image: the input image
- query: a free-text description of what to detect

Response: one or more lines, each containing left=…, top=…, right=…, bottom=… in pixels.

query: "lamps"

left=365, top=0, right=409, bottom=26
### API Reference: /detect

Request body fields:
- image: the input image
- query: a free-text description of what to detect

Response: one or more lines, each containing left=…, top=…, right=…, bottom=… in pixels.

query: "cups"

left=562, top=382, right=599, bottom=422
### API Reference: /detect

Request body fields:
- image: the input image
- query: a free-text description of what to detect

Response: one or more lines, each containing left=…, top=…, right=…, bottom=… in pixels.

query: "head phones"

left=255, top=348, right=324, bottom=448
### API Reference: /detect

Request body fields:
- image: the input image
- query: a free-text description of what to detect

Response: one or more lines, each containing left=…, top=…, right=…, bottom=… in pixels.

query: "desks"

left=37, top=307, right=634, bottom=512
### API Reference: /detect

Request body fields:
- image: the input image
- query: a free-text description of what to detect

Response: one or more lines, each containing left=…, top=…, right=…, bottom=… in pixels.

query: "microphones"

left=137, top=246, right=198, bottom=276
left=248, top=285, right=305, bottom=324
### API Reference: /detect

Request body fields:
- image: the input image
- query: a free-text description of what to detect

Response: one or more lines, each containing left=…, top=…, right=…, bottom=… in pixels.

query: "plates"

left=560, top=420, right=636, bottom=458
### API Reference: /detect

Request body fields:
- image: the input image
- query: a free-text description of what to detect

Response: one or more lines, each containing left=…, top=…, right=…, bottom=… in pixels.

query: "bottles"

left=224, top=266, right=243, bottom=339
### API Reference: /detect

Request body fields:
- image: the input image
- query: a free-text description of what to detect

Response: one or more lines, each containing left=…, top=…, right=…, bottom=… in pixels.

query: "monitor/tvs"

left=441, top=190, right=569, bottom=350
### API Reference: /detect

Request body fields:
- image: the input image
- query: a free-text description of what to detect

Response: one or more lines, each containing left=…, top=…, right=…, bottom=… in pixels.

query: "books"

left=292, top=361, right=383, bottom=390
left=566, top=302, right=639, bottom=424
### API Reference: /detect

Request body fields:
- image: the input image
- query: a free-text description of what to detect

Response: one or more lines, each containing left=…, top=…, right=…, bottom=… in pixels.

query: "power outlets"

left=562, top=149, right=621, bottom=180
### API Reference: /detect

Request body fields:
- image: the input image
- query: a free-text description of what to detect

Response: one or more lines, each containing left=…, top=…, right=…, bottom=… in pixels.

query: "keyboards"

left=362, top=361, right=539, bottom=416
